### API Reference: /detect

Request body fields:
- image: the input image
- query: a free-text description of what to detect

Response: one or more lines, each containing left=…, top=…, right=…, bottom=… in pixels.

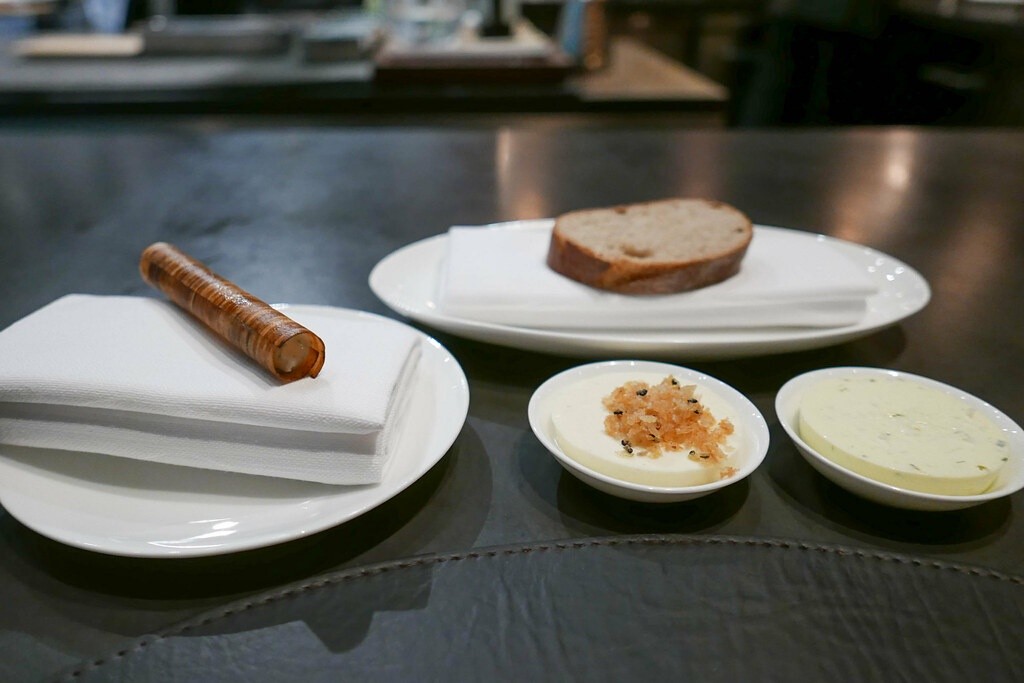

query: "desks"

left=0, top=31, right=730, bottom=128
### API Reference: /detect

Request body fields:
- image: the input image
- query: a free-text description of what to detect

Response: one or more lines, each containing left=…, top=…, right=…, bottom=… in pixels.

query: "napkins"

left=0, top=293, right=425, bottom=486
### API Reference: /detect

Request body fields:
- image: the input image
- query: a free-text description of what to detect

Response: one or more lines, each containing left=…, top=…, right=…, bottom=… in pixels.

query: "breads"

left=547, top=197, right=752, bottom=295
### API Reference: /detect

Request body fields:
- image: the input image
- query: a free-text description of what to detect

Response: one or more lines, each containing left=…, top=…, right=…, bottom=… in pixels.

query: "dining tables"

left=0, top=128, right=1024, bottom=683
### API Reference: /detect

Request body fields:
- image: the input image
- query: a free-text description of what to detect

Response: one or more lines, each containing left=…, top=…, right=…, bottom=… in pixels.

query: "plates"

left=774, top=367, right=1024, bottom=512
left=526, top=360, right=770, bottom=503
left=0, top=303, right=471, bottom=559
left=366, top=218, right=931, bottom=365
